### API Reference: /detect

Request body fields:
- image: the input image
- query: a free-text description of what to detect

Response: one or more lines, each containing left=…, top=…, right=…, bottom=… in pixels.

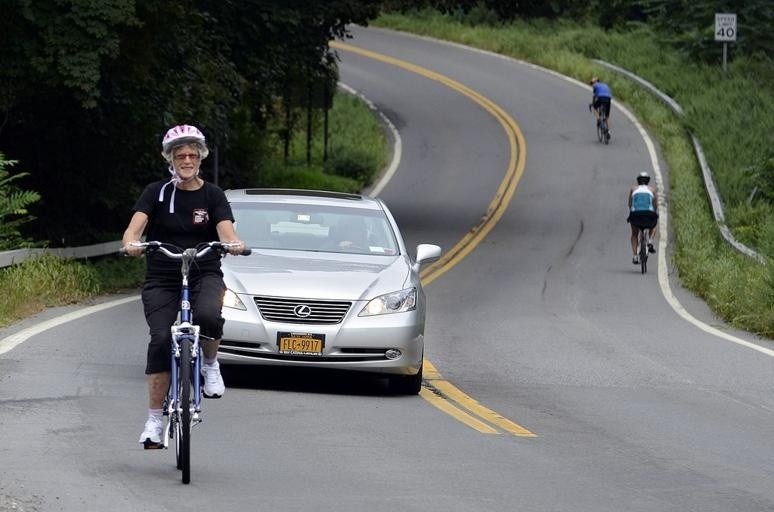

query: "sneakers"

left=139, top=411, right=169, bottom=446
left=200, top=357, right=226, bottom=399
left=647, top=240, right=656, bottom=254
left=632, top=255, right=639, bottom=264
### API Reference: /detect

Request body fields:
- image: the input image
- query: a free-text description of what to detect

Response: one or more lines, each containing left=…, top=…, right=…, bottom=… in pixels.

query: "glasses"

left=171, top=152, right=201, bottom=161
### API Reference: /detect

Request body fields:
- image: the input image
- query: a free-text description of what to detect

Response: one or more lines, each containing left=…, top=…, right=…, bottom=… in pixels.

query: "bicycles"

left=586, top=103, right=613, bottom=146
left=624, top=207, right=655, bottom=275
left=118, top=240, right=251, bottom=485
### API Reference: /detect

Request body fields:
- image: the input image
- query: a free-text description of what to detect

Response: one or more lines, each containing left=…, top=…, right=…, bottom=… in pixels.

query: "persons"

left=626, top=172, right=659, bottom=264
left=122, top=125, right=245, bottom=446
left=590, top=77, right=612, bottom=140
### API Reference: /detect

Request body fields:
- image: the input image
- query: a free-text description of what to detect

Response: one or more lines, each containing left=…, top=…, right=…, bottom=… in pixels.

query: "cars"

left=201, top=189, right=443, bottom=392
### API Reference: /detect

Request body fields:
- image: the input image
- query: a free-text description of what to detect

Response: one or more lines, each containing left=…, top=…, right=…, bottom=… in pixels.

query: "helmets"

left=160, top=122, right=211, bottom=162
left=636, top=171, right=651, bottom=181
left=589, top=77, right=599, bottom=85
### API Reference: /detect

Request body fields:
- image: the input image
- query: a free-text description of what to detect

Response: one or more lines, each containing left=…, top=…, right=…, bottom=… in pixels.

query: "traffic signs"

left=713, top=13, right=737, bottom=43
